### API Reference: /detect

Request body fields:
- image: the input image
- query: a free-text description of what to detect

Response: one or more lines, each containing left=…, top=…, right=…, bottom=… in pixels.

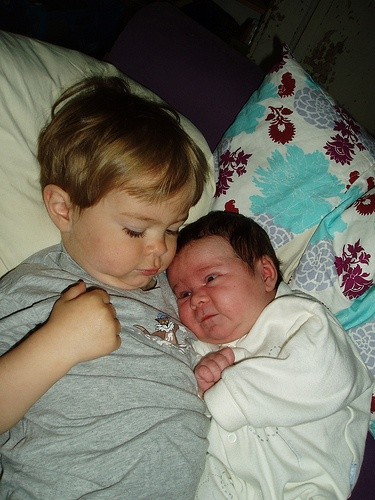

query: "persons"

left=0, top=75, right=375, bottom=500
left=165, top=210, right=374, bottom=500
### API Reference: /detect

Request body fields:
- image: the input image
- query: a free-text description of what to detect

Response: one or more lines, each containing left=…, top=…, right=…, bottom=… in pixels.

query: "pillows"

left=211, top=41, right=375, bottom=440
